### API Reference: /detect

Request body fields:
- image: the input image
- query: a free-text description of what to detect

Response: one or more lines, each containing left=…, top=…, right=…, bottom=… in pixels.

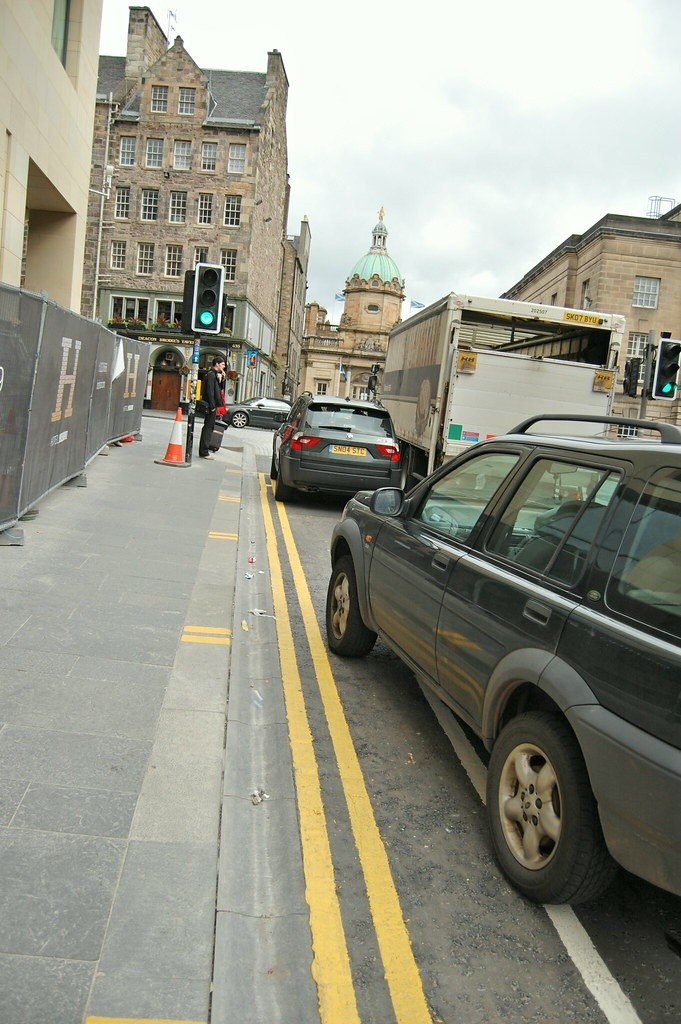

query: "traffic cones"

left=153, top=407, right=192, bottom=469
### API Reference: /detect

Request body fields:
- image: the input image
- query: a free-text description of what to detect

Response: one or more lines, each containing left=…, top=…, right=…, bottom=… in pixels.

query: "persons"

left=199, top=355, right=227, bottom=460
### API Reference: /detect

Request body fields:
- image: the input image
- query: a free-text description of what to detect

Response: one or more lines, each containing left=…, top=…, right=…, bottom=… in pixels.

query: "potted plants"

left=108, top=313, right=182, bottom=333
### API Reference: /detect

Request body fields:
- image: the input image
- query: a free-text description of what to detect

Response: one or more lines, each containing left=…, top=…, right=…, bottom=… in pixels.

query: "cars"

left=216, top=396, right=295, bottom=429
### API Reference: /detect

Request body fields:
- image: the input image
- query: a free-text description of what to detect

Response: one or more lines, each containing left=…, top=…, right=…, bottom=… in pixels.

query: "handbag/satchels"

left=193, top=399, right=209, bottom=417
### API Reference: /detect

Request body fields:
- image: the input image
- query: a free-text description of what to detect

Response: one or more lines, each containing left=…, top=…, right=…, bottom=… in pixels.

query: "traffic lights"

left=191, top=263, right=226, bottom=334
left=652, top=339, right=681, bottom=401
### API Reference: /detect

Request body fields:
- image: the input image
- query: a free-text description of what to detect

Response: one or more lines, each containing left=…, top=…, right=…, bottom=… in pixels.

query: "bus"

left=367, top=290, right=628, bottom=500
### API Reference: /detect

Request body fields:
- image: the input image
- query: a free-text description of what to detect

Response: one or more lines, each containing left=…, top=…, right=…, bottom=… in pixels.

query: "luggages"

left=208, top=414, right=229, bottom=452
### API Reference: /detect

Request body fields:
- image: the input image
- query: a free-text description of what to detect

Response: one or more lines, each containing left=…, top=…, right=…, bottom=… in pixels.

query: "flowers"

left=227, top=371, right=238, bottom=381
left=178, top=365, right=190, bottom=376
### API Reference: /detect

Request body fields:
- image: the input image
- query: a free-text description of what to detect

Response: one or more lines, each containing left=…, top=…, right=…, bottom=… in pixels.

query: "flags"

left=339, top=365, right=348, bottom=381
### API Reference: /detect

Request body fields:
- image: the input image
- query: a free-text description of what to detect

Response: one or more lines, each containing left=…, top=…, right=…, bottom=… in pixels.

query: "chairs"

left=511, top=501, right=607, bottom=581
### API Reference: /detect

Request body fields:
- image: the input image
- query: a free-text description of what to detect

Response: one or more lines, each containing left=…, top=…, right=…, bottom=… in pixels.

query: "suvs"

left=270, top=391, right=404, bottom=504
left=324, top=412, right=681, bottom=907
